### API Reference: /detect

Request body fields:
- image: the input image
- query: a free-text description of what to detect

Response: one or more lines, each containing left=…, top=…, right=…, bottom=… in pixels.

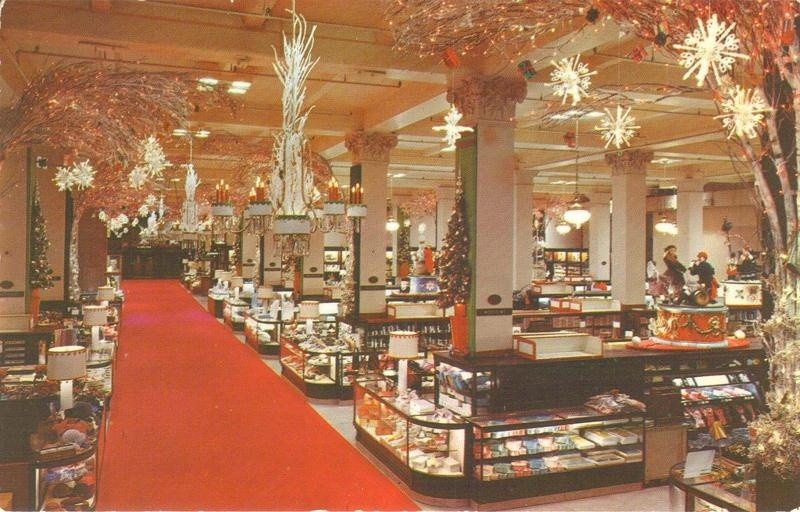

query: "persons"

left=647, top=245, right=715, bottom=305
left=726, top=245, right=754, bottom=280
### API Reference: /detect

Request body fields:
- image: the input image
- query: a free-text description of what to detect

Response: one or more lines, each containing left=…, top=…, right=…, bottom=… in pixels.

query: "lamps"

left=211, top=1, right=367, bottom=234
left=136, top=132, right=225, bottom=241
left=555, top=217, right=572, bottom=235
left=655, top=196, right=675, bottom=235
left=564, top=149, right=591, bottom=230
left=385, top=217, right=399, bottom=233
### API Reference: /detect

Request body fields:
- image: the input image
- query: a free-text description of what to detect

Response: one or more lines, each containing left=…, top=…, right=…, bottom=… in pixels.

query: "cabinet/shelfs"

left=347, top=336, right=800, bottom=512
left=0, top=263, right=138, bottom=512
left=182, top=237, right=767, bottom=398
